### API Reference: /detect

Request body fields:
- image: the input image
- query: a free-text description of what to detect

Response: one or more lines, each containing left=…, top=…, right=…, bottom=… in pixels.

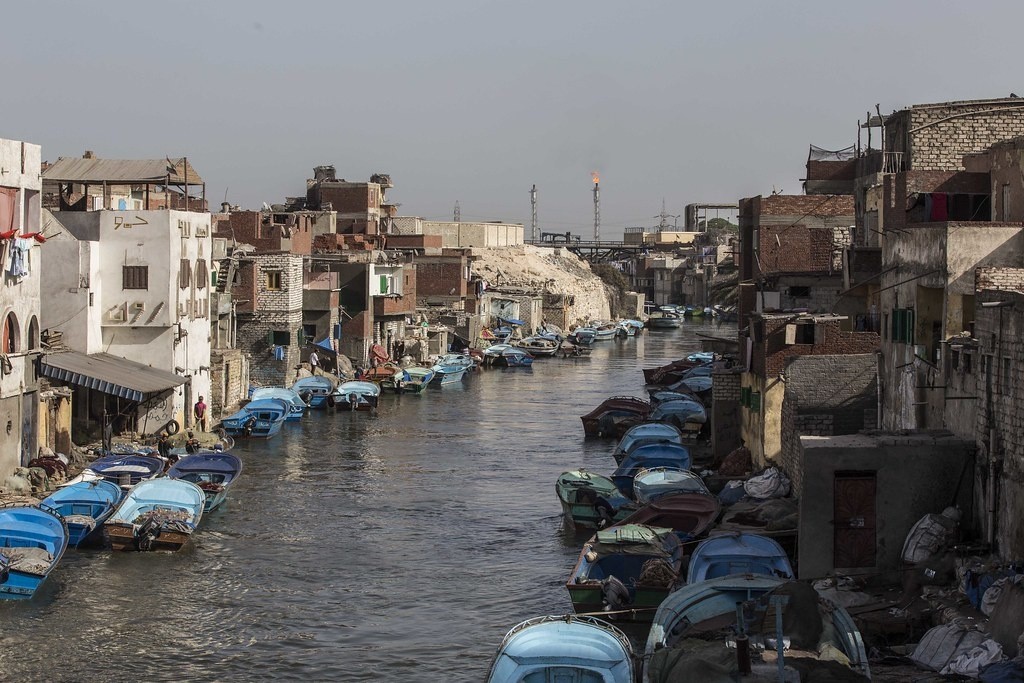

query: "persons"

left=158, top=431, right=175, bottom=457
left=194, top=396, right=206, bottom=431
left=310, top=349, right=321, bottom=375
left=186, top=432, right=201, bottom=454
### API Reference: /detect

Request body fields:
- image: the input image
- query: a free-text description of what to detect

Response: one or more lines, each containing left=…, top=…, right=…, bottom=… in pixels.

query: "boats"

left=486, top=614, right=637, bottom=683
left=429, top=357, right=475, bottom=385
left=289, top=374, right=335, bottom=411
left=35, top=479, right=122, bottom=548
left=251, top=385, right=308, bottom=419
left=580, top=339, right=740, bottom=445
left=482, top=325, right=564, bottom=367
left=102, top=477, right=206, bottom=552
left=565, top=523, right=683, bottom=623
left=0, top=501, right=69, bottom=600
left=649, top=304, right=739, bottom=328
left=163, top=452, right=243, bottom=514
left=642, top=573, right=872, bottom=683
left=89, top=454, right=165, bottom=500
left=686, top=533, right=796, bottom=585
left=553, top=421, right=798, bottom=554
left=220, top=396, right=290, bottom=438
left=567, top=320, right=643, bottom=344
left=380, top=367, right=435, bottom=394
left=327, top=379, right=382, bottom=410
